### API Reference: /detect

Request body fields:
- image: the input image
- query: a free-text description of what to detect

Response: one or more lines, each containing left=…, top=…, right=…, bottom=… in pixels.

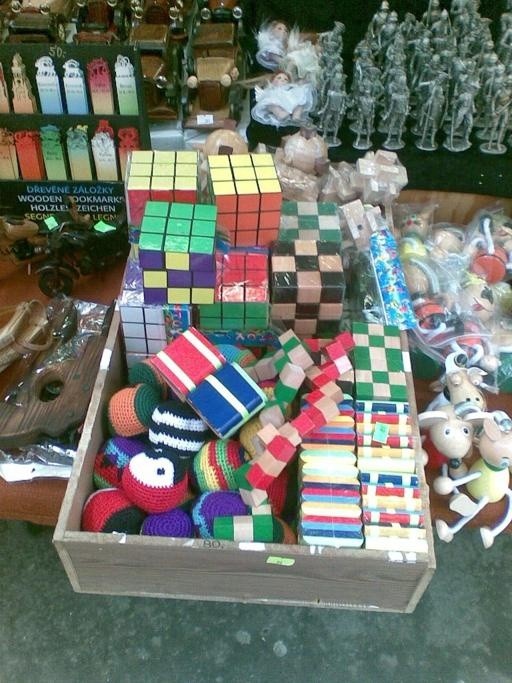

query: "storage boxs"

left=52, top=203, right=438, bottom=615
